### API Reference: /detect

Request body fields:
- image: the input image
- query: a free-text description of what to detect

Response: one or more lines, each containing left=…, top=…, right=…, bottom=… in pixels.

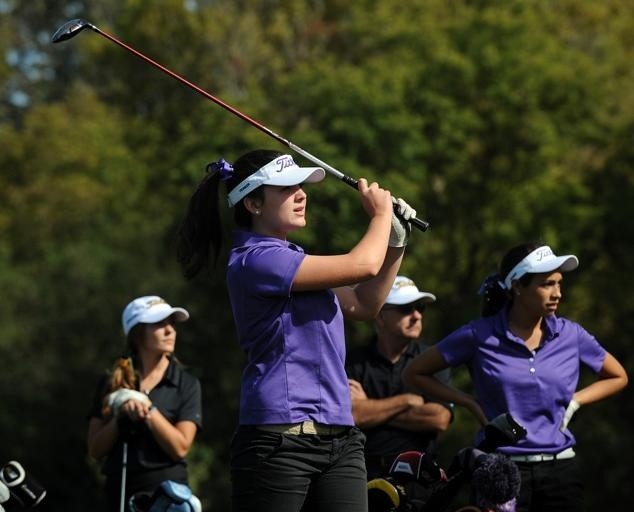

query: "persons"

left=346, top=275, right=455, bottom=512
left=402, top=242, right=630, bottom=511
left=88, top=296, right=203, bottom=511
left=174, top=148, right=416, bottom=512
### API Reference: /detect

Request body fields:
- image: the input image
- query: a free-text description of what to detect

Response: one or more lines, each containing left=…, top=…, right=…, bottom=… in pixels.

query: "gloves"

left=388, top=195, right=416, bottom=247
left=109, top=389, right=150, bottom=412
left=561, top=401, right=579, bottom=430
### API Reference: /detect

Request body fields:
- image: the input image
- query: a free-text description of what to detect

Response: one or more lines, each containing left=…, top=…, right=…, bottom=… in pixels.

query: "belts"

left=256, top=420, right=348, bottom=435
left=511, top=447, right=576, bottom=461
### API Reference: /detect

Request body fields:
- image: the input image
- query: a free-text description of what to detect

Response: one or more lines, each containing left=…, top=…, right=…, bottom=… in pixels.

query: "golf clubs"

left=50, top=18, right=429, bottom=233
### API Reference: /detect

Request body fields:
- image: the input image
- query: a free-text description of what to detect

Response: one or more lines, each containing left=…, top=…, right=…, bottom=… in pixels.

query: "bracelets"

left=143, top=405, right=157, bottom=418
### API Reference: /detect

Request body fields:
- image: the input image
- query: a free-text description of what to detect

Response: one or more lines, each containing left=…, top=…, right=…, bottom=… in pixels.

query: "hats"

left=226, top=154, right=326, bottom=206
left=385, top=277, right=435, bottom=306
left=122, top=294, right=190, bottom=334
left=500, top=246, right=579, bottom=292
